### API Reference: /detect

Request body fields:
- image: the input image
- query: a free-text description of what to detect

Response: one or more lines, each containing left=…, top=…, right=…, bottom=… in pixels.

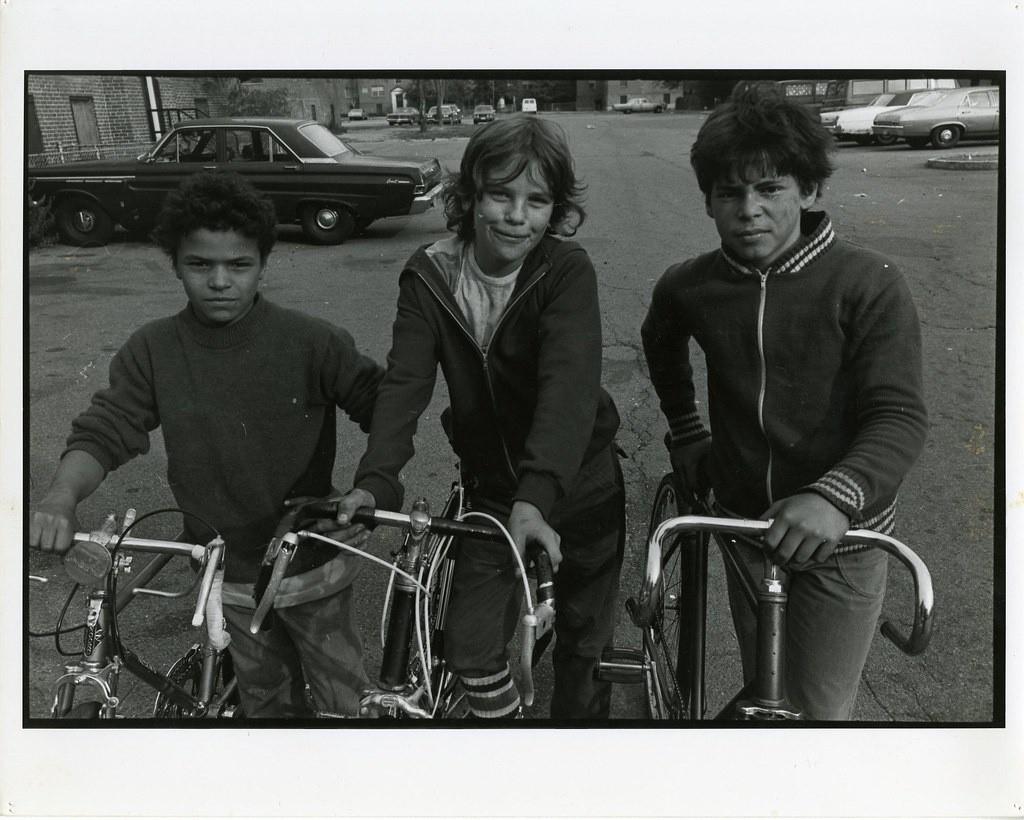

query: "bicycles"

left=595, top=427, right=939, bottom=724
left=250, top=491, right=559, bottom=720
left=28, top=507, right=245, bottom=718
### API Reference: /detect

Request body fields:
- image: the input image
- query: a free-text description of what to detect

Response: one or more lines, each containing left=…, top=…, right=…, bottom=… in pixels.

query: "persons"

left=317, top=116, right=631, bottom=719
left=638, top=86, right=926, bottom=720
left=27, top=175, right=418, bottom=718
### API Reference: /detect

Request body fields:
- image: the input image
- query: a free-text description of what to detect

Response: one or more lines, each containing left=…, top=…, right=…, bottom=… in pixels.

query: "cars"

left=818, top=89, right=952, bottom=144
left=613, top=97, right=665, bottom=114
left=29, top=116, right=449, bottom=244
left=426, top=105, right=455, bottom=125
left=348, top=109, right=369, bottom=120
left=473, top=104, right=496, bottom=124
left=387, top=107, right=420, bottom=126
left=446, top=104, right=464, bottom=124
left=872, top=85, right=1000, bottom=149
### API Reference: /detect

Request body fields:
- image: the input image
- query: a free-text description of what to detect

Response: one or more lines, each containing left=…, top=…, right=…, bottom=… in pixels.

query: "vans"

left=521, top=98, right=537, bottom=115
